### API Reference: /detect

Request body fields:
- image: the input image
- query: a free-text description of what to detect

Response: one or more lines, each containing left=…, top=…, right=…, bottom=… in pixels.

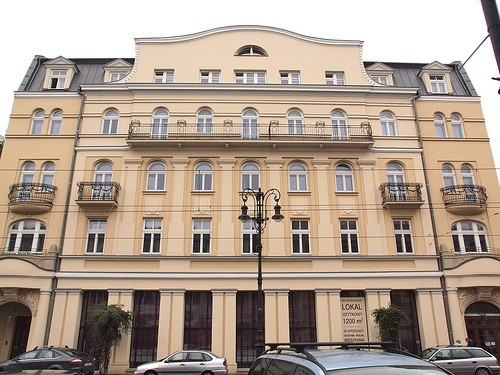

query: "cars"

left=0, top=344, right=98, bottom=375
left=133, top=349, right=228, bottom=375
left=416, top=344, right=500, bottom=375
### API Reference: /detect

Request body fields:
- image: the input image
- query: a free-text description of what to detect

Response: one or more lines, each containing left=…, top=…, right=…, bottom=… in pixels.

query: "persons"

left=465, top=338, right=474, bottom=346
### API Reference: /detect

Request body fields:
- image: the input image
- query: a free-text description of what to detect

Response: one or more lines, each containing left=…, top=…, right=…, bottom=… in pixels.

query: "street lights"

left=237, top=187, right=285, bottom=362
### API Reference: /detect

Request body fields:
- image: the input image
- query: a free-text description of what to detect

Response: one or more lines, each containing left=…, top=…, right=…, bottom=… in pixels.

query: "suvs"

left=248, top=342, right=454, bottom=375
